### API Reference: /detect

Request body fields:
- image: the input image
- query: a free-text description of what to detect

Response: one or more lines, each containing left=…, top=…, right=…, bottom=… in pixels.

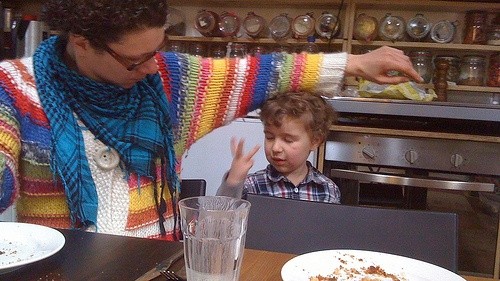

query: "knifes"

left=134, top=247, right=184, bottom=281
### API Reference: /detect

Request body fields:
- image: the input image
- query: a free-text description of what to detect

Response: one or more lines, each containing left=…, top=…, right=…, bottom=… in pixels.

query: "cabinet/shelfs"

left=163, top=0, right=500, bottom=92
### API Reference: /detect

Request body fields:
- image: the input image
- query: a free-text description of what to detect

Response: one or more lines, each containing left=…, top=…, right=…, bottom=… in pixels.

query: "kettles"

left=9, top=17, right=50, bottom=59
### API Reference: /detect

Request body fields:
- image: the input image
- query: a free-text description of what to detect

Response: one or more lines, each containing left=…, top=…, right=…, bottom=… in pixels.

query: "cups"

left=193, top=8, right=340, bottom=39
left=354, top=11, right=460, bottom=44
left=178, top=196, right=252, bottom=281
left=485, top=50, right=500, bottom=87
left=463, top=9, right=500, bottom=46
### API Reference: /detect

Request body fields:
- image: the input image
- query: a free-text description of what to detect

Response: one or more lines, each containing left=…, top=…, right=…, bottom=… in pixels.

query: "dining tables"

left=0, top=222, right=496, bottom=281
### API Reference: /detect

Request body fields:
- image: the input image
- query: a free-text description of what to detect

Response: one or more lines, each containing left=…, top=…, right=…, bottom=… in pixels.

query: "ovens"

left=317, top=119, right=500, bottom=281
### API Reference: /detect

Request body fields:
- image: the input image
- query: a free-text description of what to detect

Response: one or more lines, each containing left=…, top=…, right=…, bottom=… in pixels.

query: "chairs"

left=242, top=194, right=458, bottom=269
left=175, top=180, right=205, bottom=240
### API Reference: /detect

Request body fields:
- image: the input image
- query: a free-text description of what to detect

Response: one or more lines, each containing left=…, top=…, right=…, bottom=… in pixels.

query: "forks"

left=158, top=269, right=187, bottom=281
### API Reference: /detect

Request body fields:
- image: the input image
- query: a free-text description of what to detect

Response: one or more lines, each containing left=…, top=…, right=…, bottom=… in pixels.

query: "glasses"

left=92, top=34, right=171, bottom=72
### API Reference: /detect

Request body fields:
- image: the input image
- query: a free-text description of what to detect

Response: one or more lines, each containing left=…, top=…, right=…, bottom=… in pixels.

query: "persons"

left=1, top=0, right=423, bottom=241
left=215, top=89, right=340, bottom=204
left=183, top=198, right=200, bottom=236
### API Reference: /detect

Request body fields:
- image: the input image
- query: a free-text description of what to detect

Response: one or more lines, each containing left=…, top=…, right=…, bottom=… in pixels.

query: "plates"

left=0, top=221, right=66, bottom=275
left=280, top=247, right=468, bottom=281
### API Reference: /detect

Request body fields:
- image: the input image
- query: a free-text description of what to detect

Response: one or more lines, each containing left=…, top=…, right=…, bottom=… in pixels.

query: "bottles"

left=300, top=36, right=319, bottom=54
left=406, top=50, right=433, bottom=84
left=457, top=52, right=487, bottom=86
left=433, top=55, right=460, bottom=85
left=167, top=41, right=292, bottom=59
left=2, top=7, right=14, bottom=28
